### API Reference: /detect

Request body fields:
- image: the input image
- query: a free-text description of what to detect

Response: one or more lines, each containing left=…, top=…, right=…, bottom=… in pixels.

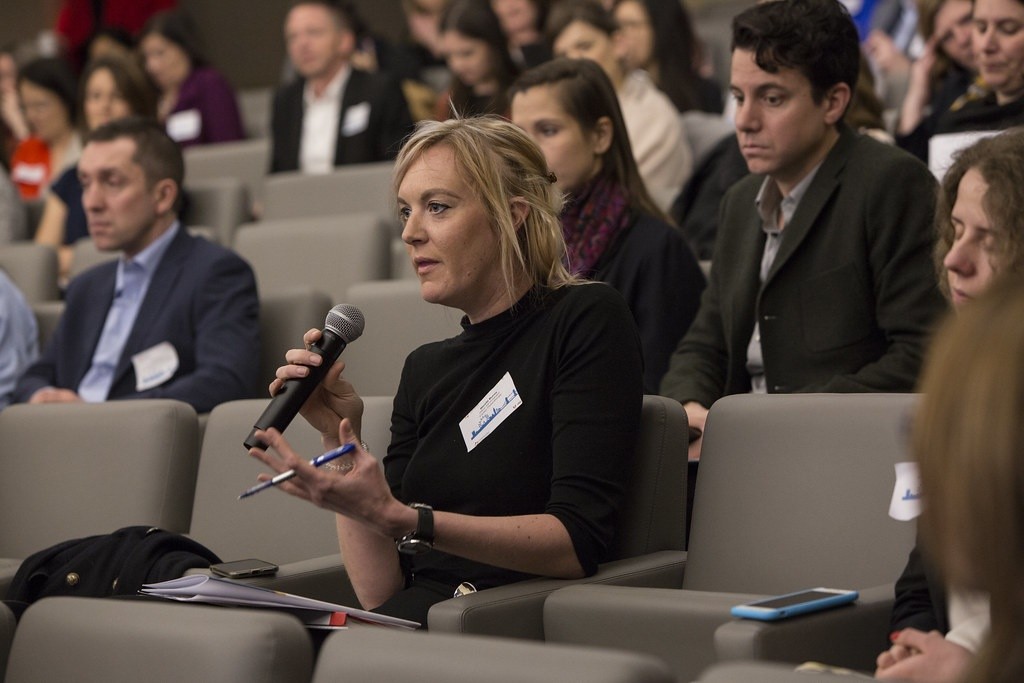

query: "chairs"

left=0, top=90, right=1024, bottom=683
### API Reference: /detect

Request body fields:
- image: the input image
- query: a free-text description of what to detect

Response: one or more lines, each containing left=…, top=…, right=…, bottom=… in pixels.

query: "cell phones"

left=209, top=558, right=279, bottom=579
left=731, top=587, right=859, bottom=621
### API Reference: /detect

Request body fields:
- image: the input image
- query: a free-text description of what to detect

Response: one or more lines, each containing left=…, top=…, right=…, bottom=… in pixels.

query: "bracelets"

left=324, top=440, right=369, bottom=472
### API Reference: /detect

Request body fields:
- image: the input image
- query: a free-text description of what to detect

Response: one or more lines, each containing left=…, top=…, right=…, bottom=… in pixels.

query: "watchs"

left=393, top=502, right=436, bottom=557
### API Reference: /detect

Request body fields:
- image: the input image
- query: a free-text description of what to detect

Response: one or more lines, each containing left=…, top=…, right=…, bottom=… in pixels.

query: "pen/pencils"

left=237, top=444, right=357, bottom=499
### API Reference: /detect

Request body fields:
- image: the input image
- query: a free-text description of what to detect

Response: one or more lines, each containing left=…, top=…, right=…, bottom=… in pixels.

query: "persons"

left=0, top=269, right=41, bottom=406
left=268, top=0, right=1024, bottom=264
left=8, top=114, right=264, bottom=418
left=247, top=93, right=644, bottom=666
left=501, top=59, right=708, bottom=394
left=870, top=125, right=1024, bottom=683
left=3, top=2, right=245, bottom=301
left=656, top=1, right=954, bottom=549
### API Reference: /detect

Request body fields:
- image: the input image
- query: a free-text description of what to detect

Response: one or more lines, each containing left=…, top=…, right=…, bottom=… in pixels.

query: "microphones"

left=243, top=304, right=365, bottom=452
